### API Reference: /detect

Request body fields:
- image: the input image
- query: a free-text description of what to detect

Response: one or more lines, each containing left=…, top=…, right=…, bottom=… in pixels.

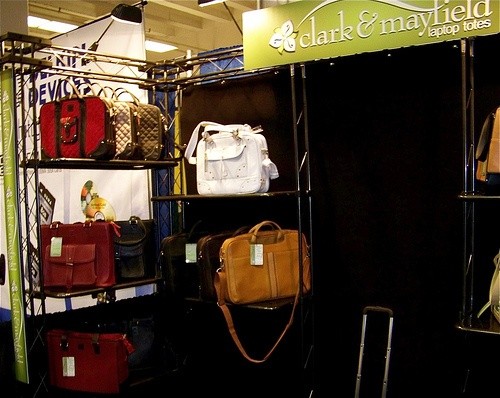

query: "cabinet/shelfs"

left=0, top=32, right=500, bottom=391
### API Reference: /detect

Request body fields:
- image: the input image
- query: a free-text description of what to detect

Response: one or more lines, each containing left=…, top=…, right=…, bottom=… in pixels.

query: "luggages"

left=354, top=307, right=393, bottom=398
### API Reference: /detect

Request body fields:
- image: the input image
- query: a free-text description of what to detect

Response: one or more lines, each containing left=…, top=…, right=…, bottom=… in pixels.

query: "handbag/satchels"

left=476, top=251, right=500, bottom=324
left=40, top=219, right=120, bottom=290
left=475, top=107, right=500, bottom=185
left=198, top=224, right=253, bottom=299
left=46, top=329, right=136, bottom=392
left=214, top=220, right=311, bottom=363
left=40, top=79, right=118, bottom=159
left=184, top=120, right=280, bottom=194
left=84, top=82, right=142, bottom=159
left=157, top=221, right=203, bottom=287
left=111, top=87, right=169, bottom=159
left=96, top=215, right=157, bottom=281
